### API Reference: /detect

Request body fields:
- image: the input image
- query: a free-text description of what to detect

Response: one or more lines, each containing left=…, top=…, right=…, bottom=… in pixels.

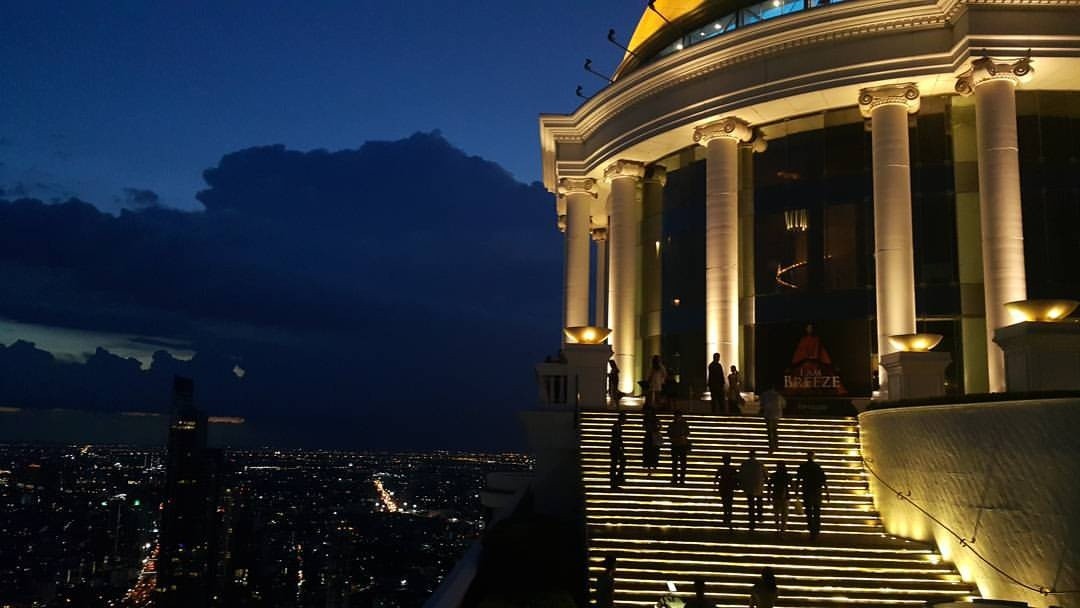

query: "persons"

left=608, top=352, right=744, bottom=416
left=751, top=566, right=779, bottom=608
left=717, top=449, right=830, bottom=535
left=610, top=412, right=626, bottom=487
left=760, top=382, right=785, bottom=449
left=683, top=576, right=717, bottom=608
left=668, top=410, right=689, bottom=486
left=642, top=407, right=661, bottom=472
